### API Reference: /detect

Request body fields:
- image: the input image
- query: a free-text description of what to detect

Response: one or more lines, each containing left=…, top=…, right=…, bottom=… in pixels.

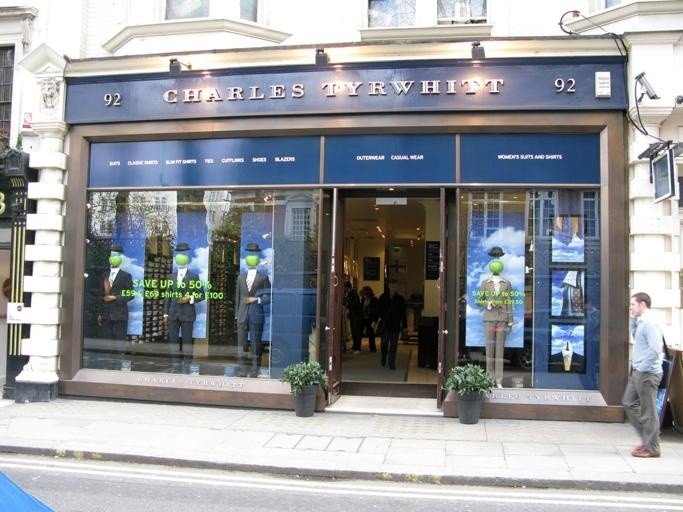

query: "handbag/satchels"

left=658, top=356, right=674, bottom=389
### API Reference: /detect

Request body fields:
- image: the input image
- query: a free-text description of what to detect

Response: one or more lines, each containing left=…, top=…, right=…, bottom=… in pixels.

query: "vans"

left=248, top=289, right=327, bottom=367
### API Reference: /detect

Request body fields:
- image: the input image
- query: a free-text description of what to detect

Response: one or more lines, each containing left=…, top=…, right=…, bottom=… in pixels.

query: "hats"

left=108, top=245, right=124, bottom=252
left=488, top=247, right=505, bottom=257
left=174, top=242, right=191, bottom=251
left=244, top=243, right=262, bottom=252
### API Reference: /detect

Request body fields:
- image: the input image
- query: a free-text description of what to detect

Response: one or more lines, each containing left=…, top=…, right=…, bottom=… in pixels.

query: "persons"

left=162, top=253, right=206, bottom=374
left=476, top=261, right=514, bottom=388
left=344, top=277, right=409, bottom=371
left=622, top=292, right=664, bottom=458
left=234, top=255, right=271, bottom=378
left=96, top=255, right=136, bottom=370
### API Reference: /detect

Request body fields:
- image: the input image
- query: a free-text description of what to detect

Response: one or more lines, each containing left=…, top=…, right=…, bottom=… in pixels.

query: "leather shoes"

left=632, top=445, right=662, bottom=458
left=380, top=359, right=398, bottom=371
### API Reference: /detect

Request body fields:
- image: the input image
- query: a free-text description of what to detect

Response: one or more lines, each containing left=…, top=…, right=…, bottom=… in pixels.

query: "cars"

left=459, top=275, right=600, bottom=369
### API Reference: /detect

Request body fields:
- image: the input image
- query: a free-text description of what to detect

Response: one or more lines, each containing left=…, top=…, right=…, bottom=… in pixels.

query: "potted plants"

left=443, top=363, right=498, bottom=425
left=280, top=363, right=328, bottom=417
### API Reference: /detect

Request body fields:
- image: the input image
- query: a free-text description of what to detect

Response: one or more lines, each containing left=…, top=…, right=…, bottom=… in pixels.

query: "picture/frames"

left=547, top=214, right=588, bottom=374
left=364, top=257, right=380, bottom=281
left=427, top=241, right=440, bottom=281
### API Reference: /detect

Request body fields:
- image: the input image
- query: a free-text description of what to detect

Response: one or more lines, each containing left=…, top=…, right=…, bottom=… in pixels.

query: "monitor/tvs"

left=652, top=149, right=676, bottom=204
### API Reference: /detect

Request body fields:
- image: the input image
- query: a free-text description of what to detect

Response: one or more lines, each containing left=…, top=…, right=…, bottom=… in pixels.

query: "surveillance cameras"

left=635, top=71, right=660, bottom=101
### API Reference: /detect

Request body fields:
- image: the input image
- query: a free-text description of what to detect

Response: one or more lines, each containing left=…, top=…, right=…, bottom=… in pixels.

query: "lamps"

left=470, top=42, right=486, bottom=62
left=313, top=49, right=329, bottom=69
left=169, top=58, right=192, bottom=78
